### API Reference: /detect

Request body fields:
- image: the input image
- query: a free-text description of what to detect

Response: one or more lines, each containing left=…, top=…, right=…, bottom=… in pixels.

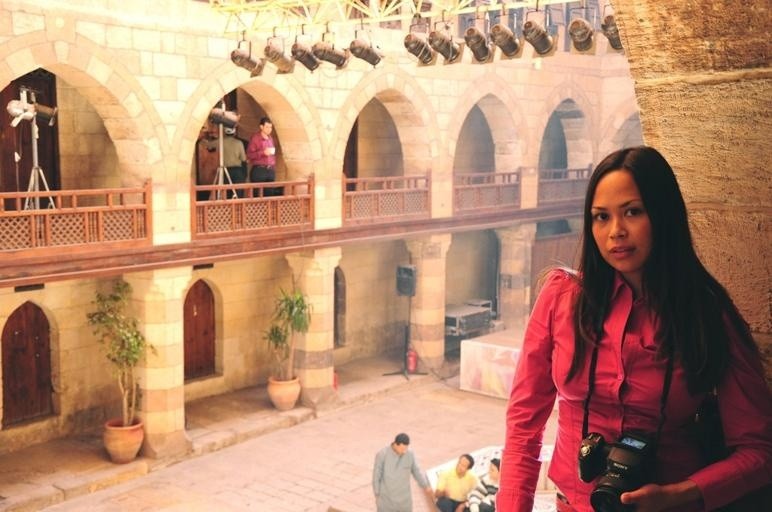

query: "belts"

left=254, top=164, right=273, bottom=170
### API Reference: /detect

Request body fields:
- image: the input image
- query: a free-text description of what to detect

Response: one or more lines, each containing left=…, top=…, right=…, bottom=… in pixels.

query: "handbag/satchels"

left=687, top=402, right=770, bottom=512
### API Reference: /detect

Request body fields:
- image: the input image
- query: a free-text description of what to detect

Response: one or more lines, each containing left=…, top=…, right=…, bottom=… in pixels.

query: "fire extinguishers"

left=334, top=369, right=339, bottom=390
left=406, top=351, right=417, bottom=374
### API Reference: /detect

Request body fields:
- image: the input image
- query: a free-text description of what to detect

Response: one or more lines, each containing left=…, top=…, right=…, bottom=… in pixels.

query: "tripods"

left=383, top=301, right=428, bottom=381
left=209, top=128, right=237, bottom=199
left=20, top=126, right=56, bottom=210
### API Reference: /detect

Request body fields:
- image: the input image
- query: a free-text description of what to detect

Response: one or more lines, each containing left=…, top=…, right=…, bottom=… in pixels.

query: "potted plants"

left=261, top=284, right=313, bottom=411
left=84, top=276, right=160, bottom=465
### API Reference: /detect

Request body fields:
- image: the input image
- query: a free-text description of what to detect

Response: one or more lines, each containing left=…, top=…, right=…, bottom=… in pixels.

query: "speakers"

left=396, top=265, right=416, bottom=296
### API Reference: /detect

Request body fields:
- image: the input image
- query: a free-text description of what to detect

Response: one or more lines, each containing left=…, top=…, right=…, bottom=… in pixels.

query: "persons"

left=246, top=117, right=277, bottom=197
left=467, top=457, right=501, bottom=512
left=206, top=125, right=246, bottom=199
left=371, top=431, right=434, bottom=512
left=494, top=145, right=771, bottom=511
left=434, top=453, right=479, bottom=512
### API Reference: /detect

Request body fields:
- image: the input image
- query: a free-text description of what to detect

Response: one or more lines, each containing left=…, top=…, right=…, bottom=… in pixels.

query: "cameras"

left=576, top=428, right=658, bottom=512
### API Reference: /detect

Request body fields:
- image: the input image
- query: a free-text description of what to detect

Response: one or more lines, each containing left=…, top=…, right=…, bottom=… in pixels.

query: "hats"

left=225, top=127, right=236, bottom=134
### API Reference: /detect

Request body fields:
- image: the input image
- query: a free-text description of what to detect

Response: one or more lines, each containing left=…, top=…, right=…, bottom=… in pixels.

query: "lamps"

left=7, top=85, right=59, bottom=209
left=209, top=99, right=241, bottom=200
left=223, top=0, right=622, bottom=81
